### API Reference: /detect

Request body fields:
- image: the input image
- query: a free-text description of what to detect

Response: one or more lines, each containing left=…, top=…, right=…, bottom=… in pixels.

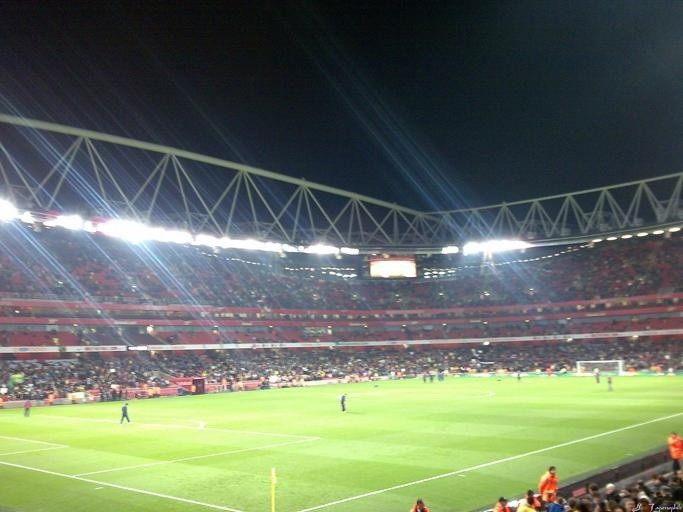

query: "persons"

left=408, top=432, right=683, bottom=512
left=1, top=240, right=683, bottom=423
